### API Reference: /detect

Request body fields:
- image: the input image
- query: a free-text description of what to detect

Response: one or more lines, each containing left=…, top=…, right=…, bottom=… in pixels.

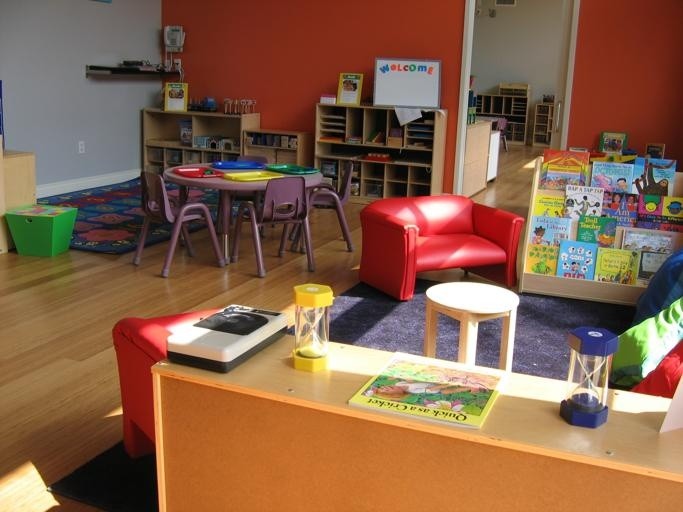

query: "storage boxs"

left=5, top=202, right=78, bottom=255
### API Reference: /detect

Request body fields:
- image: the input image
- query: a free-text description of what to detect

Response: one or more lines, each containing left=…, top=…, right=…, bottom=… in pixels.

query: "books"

left=348, top=350, right=512, bottom=430
left=525, top=130, right=683, bottom=289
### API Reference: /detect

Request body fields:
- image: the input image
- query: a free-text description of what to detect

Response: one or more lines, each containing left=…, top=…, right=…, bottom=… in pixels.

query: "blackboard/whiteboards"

left=373, top=58, right=442, bottom=109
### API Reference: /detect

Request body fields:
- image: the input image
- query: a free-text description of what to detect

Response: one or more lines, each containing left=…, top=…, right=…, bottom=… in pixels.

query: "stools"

left=424, top=279, right=520, bottom=372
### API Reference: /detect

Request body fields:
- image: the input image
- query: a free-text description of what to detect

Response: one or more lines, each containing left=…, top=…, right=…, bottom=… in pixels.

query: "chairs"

left=133, top=155, right=354, bottom=278
left=113, top=306, right=225, bottom=458
left=360, top=193, right=526, bottom=300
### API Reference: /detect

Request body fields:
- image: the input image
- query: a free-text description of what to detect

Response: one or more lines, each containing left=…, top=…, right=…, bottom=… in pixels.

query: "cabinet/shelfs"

left=0, top=152, right=35, bottom=253
left=517, top=156, right=683, bottom=306
left=144, top=103, right=307, bottom=172
left=462, top=82, right=553, bottom=198
left=151, top=332, right=683, bottom=512
left=315, top=104, right=449, bottom=202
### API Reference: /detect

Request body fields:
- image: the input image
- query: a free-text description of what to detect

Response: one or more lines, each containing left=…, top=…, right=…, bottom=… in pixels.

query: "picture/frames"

left=495, top=0, right=516, bottom=7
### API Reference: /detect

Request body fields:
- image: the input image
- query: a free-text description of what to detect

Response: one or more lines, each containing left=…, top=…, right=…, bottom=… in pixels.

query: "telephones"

left=164, top=26, right=183, bottom=47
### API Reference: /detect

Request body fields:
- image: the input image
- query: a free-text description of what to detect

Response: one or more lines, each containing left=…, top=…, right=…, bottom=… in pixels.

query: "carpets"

left=38, top=174, right=251, bottom=255
left=43, top=269, right=634, bottom=511
